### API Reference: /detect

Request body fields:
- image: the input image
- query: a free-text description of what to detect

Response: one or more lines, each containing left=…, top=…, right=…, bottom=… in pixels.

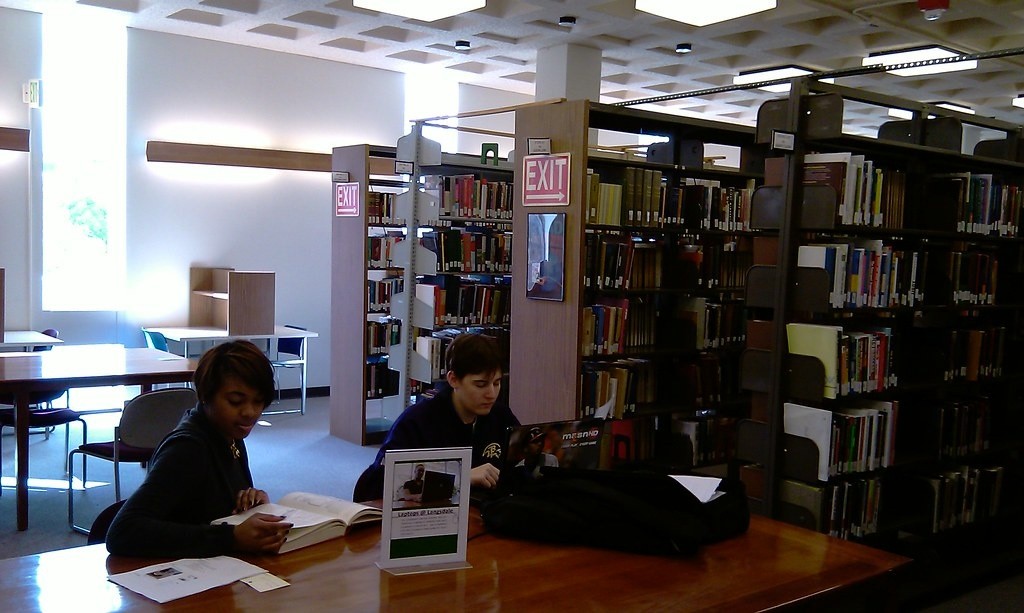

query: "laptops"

left=469, top=416, right=606, bottom=509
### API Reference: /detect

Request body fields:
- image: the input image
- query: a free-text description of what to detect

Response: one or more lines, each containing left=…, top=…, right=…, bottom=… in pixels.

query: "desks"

left=0, top=348, right=200, bottom=531
left=145, top=325, right=319, bottom=417
left=1, top=502, right=910, bottom=613
left=0, top=331, right=64, bottom=351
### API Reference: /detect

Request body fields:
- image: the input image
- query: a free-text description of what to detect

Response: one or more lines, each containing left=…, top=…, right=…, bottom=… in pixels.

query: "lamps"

left=732, top=65, right=836, bottom=93
left=1012, top=93, right=1024, bottom=109
left=863, top=45, right=978, bottom=78
left=888, top=100, right=976, bottom=119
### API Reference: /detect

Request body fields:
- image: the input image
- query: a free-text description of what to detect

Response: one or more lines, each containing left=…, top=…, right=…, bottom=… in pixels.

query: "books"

left=210, top=492, right=382, bottom=554
left=368, top=153, right=1024, bottom=541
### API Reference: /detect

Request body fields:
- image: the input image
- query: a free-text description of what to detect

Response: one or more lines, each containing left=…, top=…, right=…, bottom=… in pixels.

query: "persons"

left=105, top=340, right=294, bottom=557
left=403, top=464, right=425, bottom=501
left=515, top=428, right=558, bottom=468
left=374, top=334, right=523, bottom=493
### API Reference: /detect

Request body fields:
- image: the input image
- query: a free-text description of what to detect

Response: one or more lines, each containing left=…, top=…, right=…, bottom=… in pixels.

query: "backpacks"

left=479, top=465, right=750, bottom=556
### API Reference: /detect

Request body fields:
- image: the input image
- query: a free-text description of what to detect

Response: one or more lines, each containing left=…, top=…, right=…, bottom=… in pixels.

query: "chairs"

left=0, top=381, right=88, bottom=491
left=267, top=325, right=309, bottom=403
left=141, top=324, right=203, bottom=390
left=68, top=387, right=198, bottom=535
left=35, top=328, right=59, bottom=350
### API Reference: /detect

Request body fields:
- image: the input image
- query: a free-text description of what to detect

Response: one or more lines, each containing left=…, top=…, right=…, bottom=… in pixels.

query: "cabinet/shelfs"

left=513, top=100, right=757, bottom=420
left=753, top=76, right=1024, bottom=533
left=189, top=267, right=275, bottom=335
left=392, top=125, right=515, bottom=417
left=331, top=143, right=404, bottom=444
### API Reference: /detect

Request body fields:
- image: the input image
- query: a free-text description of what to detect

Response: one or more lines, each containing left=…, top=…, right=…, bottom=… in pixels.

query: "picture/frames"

left=526, top=212, right=565, bottom=302
